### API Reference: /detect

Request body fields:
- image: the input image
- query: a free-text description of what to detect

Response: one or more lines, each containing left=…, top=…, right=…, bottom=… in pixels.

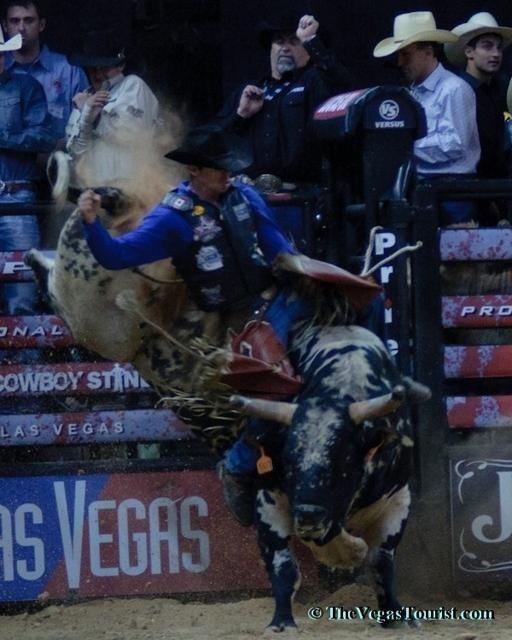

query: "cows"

left=22, top=112, right=431, bottom=637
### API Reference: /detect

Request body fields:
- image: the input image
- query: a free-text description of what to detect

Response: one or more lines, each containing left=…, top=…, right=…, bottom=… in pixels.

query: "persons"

left=0, top=0, right=511, bottom=528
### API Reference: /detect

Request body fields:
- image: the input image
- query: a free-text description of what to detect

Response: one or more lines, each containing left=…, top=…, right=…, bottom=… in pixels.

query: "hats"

left=164, top=125, right=254, bottom=171
left=444, top=10, right=512, bottom=67
left=67, top=30, right=129, bottom=69
left=0, top=24, right=24, bottom=56
left=255, top=6, right=332, bottom=50
left=370, top=9, right=460, bottom=58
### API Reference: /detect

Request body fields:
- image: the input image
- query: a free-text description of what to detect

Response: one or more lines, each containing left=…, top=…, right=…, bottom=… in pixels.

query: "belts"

left=240, top=172, right=306, bottom=195
left=205, top=284, right=283, bottom=312
left=0, top=175, right=40, bottom=197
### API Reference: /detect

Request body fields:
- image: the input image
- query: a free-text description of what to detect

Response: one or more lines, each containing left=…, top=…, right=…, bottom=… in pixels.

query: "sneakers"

left=399, top=370, right=434, bottom=408
left=215, top=456, right=259, bottom=528
